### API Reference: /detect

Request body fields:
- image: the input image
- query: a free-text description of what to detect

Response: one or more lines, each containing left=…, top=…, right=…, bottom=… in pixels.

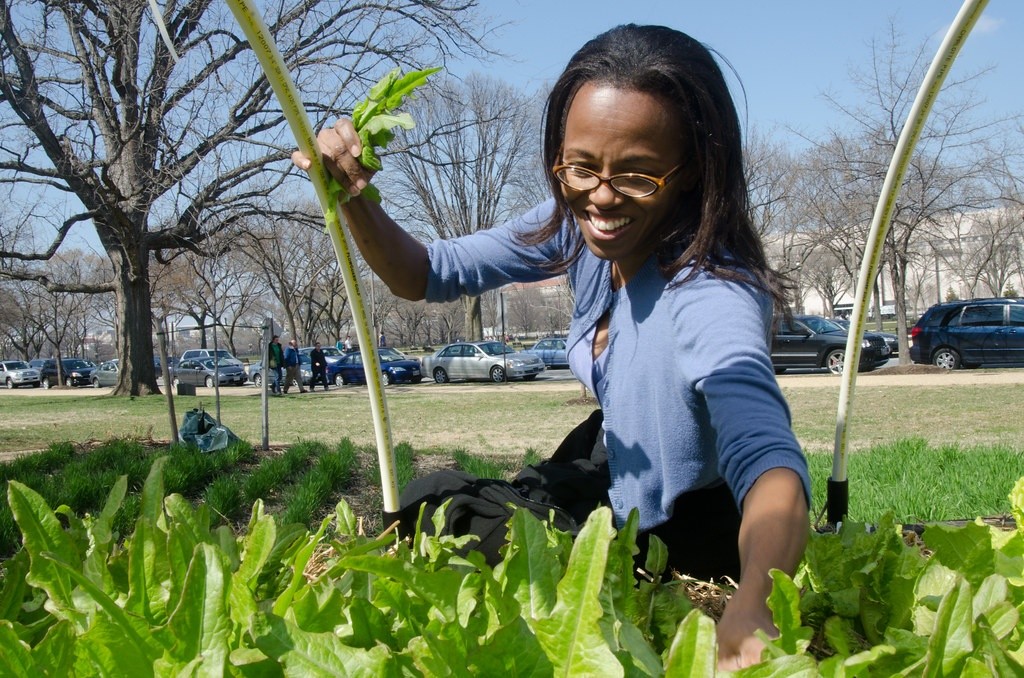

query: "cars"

left=89, top=361, right=120, bottom=387
left=302, top=347, right=347, bottom=365
left=154, top=355, right=181, bottom=378
left=420, top=340, right=546, bottom=383
left=40, top=358, right=91, bottom=389
left=0, top=360, right=41, bottom=389
left=326, top=349, right=421, bottom=386
left=29, top=359, right=47, bottom=373
left=519, top=338, right=567, bottom=367
left=248, top=351, right=331, bottom=386
left=346, top=347, right=421, bottom=367
left=910, top=298, right=1024, bottom=369
left=85, top=360, right=96, bottom=367
left=827, top=318, right=899, bottom=359
left=169, top=357, right=245, bottom=388
left=770, top=314, right=890, bottom=375
left=180, top=349, right=245, bottom=372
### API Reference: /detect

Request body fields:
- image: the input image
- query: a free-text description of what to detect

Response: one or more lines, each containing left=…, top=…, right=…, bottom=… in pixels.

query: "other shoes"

left=324, top=387, right=331, bottom=391
left=270, top=385, right=276, bottom=393
left=276, top=392, right=281, bottom=395
left=310, top=388, right=316, bottom=392
left=284, top=391, right=287, bottom=393
left=301, top=390, right=308, bottom=393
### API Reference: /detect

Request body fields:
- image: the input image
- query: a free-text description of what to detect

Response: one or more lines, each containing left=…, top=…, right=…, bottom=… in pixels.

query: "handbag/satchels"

left=397, top=468, right=577, bottom=564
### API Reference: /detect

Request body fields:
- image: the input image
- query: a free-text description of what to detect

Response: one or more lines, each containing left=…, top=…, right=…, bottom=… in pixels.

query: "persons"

left=504, top=334, right=509, bottom=345
left=345, top=336, right=352, bottom=353
left=291, top=22, right=811, bottom=665
left=380, top=331, right=386, bottom=347
left=269, top=335, right=283, bottom=397
left=336, top=338, right=342, bottom=351
left=309, top=342, right=329, bottom=392
left=283, top=340, right=307, bottom=393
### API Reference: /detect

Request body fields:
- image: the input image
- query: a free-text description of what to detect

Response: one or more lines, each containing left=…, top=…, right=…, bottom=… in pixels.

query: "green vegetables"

left=325, top=66, right=443, bottom=205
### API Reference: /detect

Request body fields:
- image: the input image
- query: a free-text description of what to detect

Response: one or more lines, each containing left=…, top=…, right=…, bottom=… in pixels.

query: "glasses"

left=553, top=143, right=697, bottom=198
